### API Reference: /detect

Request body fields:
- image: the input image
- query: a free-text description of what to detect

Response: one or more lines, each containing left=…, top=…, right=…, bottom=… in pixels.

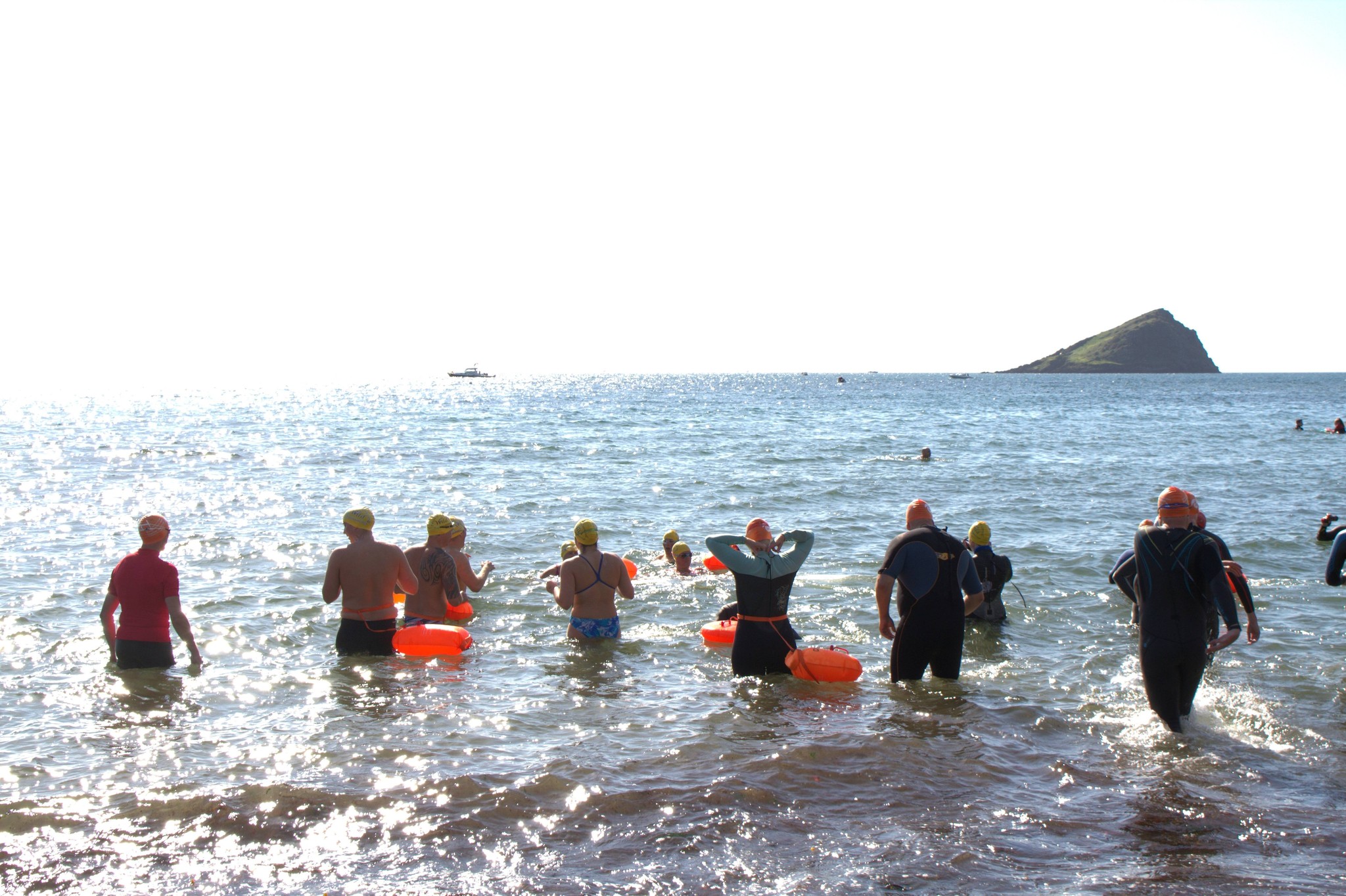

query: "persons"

left=1295, top=419, right=1303, bottom=430
left=445, top=517, right=495, bottom=602
left=1317, top=513, right=1346, bottom=586
left=876, top=499, right=984, bottom=685
left=961, top=520, right=1012, bottom=626
left=1325, top=418, right=1346, bottom=434
left=705, top=517, right=815, bottom=677
left=1109, top=487, right=1260, bottom=732
left=654, top=530, right=707, bottom=576
left=917, top=446, right=931, bottom=460
left=98, top=516, right=204, bottom=670
left=322, top=508, right=419, bottom=656
left=540, top=520, right=635, bottom=642
left=393, top=514, right=467, bottom=627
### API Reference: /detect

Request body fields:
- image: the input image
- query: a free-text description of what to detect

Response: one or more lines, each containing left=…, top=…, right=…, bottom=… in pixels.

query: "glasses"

left=660, top=538, right=680, bottom=544
left=427, top=522, right=456, bottom=529
left=964, top=535, right=970, bottom=542
left=164, top=528, right=171, bottom=534
left=562, top=545, right=579, bottom=551
left=675, top=552, right=693, bottom=558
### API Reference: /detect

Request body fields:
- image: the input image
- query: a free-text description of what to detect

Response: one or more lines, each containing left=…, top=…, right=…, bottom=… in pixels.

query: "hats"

left=448, top=515, right=465, bottom=539
left=905, top=498, right=933, bottom=523
left=1157, top=486, right=1190, bottom=518
left=574, top=518, right=598, bottom=545
left=1184, top=491, right=1199, bottom=516
left=427, top=512, right=455, bottom=536
left=745, top=517, right=772, bottom=542
left=1138, top=519, right=1153, bottom=527
left=561, top=540, right=578, bottom=557
left=343, top=505, right=375, bottom=531
left=138, top=512, right=170, bottom=544
left=1197, top=511, right=1207, bottom=529
left=671, top=541, right=691, bottom=560
left=663, top=530, right=679, bottom=543
left=969, top=520, right=991, bottom=546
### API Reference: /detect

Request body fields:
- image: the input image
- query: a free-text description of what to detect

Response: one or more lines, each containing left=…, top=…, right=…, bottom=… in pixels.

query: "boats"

left=801, top=372, right=808, bottom=375
left=837, top=377, right=846, bottom=383
left=949, top=373, right=974, bottom=379
left=868, top=371, right=878, bottom=373
left=447, top=368, right=496, bottom=378
